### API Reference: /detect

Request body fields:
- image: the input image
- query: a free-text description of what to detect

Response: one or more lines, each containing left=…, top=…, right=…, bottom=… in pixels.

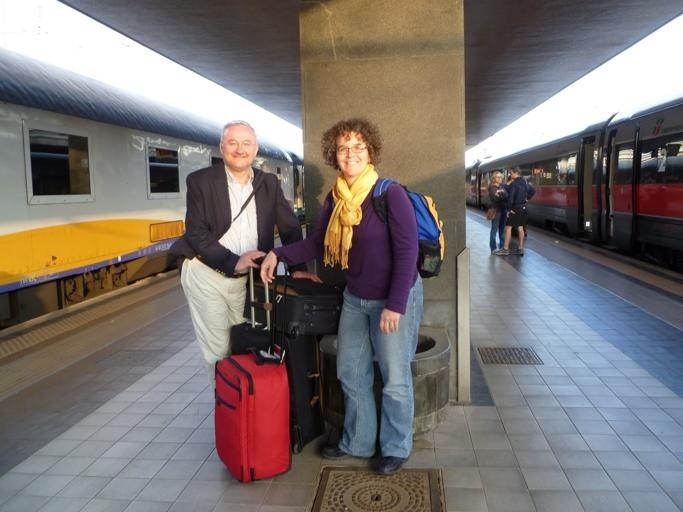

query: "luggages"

left=243, top=274, right=343, bottom=334
left=229, top=258, right=323, bottom=454
left=215, top=264, right=291, bottom=483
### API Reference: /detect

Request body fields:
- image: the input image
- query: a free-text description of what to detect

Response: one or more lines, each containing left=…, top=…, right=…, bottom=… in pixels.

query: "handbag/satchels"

left=487, top=208, right=496, bottom=219
left=168, top=239, right=183, bottom=267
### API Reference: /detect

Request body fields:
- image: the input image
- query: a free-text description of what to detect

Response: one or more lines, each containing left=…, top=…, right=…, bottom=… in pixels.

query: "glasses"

left=339, top=143, right=366, bottom=155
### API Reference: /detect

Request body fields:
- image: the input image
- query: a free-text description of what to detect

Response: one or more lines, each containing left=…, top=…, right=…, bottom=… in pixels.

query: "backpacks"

left=373, top=178, right=444, bottom=279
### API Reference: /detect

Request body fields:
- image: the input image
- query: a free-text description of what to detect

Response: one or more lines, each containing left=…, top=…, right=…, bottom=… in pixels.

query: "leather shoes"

left=321, top=440, right=347, bottom=459
left=379, top=455, right=407, bottom=474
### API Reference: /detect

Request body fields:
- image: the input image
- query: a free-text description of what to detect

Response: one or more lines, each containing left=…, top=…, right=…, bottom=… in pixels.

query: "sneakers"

left=511, top=248, right=523, bottom=255
left=496, top=249, right=510, bottom=255
left=491, top=248, right=498, bottom=255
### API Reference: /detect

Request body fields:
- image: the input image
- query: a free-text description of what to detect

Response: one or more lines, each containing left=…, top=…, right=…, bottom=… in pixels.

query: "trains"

left=464, top=94, right=682, bottom=279
left=0, top=44, right=307, bottom=333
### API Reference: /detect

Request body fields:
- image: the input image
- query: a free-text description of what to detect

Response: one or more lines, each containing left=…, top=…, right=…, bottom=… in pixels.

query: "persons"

left=166, top=118, right=324, bottom=372
left=502, top=175, right=529, bottom=237
left=486, top=170, right=510, bottom=256
left=257, top=116, right=424, bottom=479
left=492, top=166, right=536, bottom=256
